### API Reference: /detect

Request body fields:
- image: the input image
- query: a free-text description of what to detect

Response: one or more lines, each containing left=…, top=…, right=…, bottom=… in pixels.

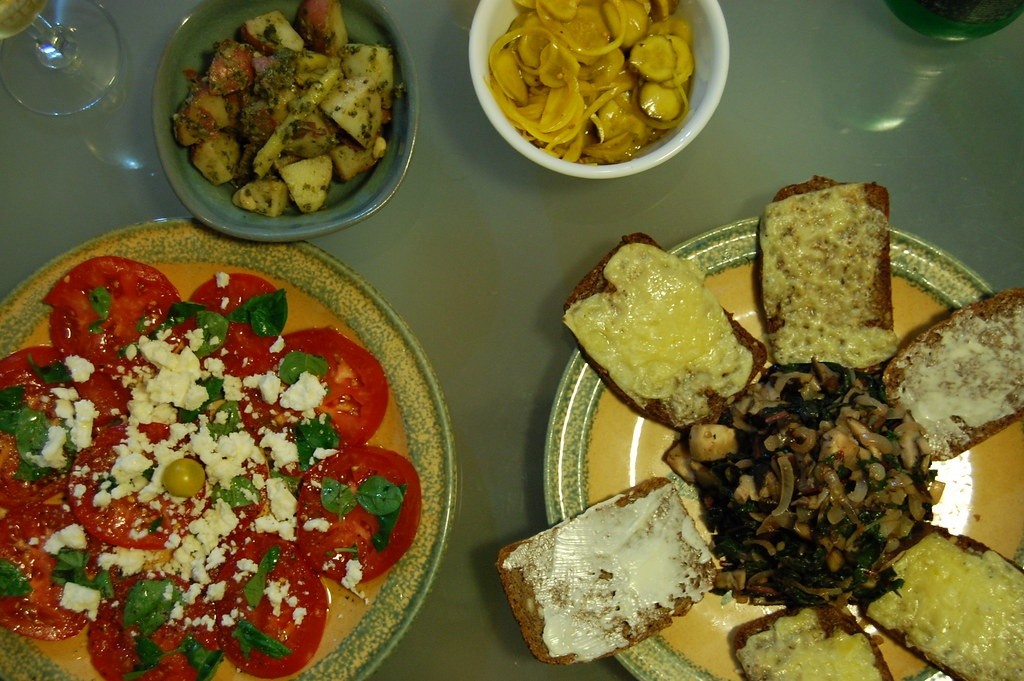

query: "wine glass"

left=0, top=0, right=120, bottom=116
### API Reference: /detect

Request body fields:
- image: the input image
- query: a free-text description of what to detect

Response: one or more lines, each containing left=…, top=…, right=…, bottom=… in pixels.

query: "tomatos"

left=0, top=257, right=422, bottom=678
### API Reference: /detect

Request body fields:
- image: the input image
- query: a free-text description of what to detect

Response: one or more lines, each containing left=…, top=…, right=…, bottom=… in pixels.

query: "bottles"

left=886, top=0, right=1024, bottom=42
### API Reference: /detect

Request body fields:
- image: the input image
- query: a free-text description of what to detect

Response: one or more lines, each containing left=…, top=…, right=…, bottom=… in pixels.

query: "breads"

left=492, top=179, right=1024, bottom=681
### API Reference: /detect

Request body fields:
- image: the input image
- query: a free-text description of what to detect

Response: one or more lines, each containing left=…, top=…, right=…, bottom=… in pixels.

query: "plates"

left=546, top=219, right=1024, bottom=681
left=0, top=219, right=457, bottom=681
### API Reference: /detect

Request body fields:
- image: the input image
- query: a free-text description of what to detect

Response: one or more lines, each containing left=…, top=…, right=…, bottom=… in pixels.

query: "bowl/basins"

left=153, top=0, right=418, bottom=242
left=467, top=0, right=731, bottom=180
